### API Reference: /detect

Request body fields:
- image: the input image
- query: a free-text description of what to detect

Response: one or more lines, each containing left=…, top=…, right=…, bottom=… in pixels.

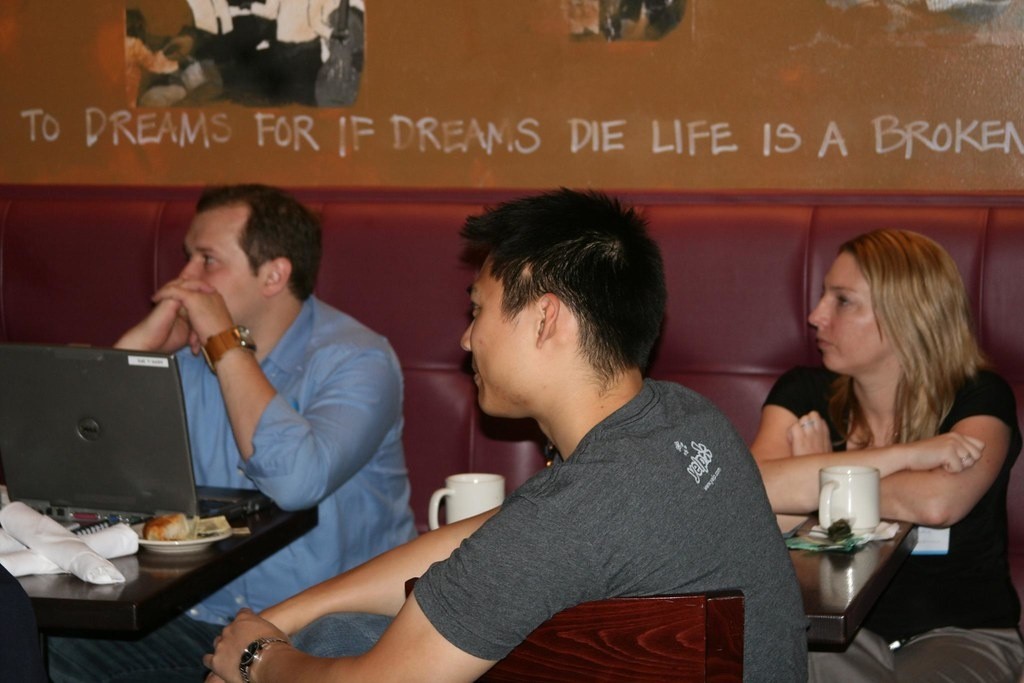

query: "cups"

left=819, top=465, right=881, bottom=532
left=427, top=473, right=506, bottom=531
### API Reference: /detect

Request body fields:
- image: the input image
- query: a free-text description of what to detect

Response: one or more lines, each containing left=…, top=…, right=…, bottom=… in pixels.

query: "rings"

left=961, top=453, right=972, bottom=463
left=801, top=420, right=814, bottom=428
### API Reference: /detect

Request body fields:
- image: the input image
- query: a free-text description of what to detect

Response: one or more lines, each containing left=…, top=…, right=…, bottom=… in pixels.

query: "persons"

left=49, top=183, right=422, bottom=683
left=207, top=186, right=811, bottom=683
left=748, top=226, right=1024, bottom=683
left=126, top=2, right=364, bottom=108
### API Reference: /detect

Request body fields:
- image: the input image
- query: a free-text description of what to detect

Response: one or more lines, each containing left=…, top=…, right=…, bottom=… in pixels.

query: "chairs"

left=403, top=576, right=745, bottom=683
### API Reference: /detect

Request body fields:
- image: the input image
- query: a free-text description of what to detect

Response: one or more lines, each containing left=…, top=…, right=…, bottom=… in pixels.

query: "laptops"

left=0, top=343, right=275, bottom=527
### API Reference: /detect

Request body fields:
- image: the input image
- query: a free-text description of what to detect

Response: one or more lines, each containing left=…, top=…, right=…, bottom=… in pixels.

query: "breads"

left=142, top=513, right=230, bottom=541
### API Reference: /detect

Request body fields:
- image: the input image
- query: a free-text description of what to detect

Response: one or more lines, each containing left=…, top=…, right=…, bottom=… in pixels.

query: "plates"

left=131, top=522, right=234, bottom=555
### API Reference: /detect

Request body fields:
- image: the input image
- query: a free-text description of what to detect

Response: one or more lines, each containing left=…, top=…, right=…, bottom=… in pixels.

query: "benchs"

left=0, top=182, right=1024, bottom=637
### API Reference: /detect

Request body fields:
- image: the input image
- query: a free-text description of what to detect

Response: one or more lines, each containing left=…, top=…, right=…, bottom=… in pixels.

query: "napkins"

left=0, top=522, right=139, bottom=579
left=810, top=520, right=900, bottom=540
left=0, top=501, right=126, bottom=585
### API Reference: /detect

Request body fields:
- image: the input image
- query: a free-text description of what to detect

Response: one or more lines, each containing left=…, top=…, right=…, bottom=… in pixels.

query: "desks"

left=774, top=511, right=920, bottom=654
left=0, top=485, right=320, bottom=673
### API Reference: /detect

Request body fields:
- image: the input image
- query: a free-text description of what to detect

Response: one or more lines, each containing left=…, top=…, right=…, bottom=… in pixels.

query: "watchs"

left=201, top=325, right=258, bottom=375
left=239, top=635, right=291, bottom=683
